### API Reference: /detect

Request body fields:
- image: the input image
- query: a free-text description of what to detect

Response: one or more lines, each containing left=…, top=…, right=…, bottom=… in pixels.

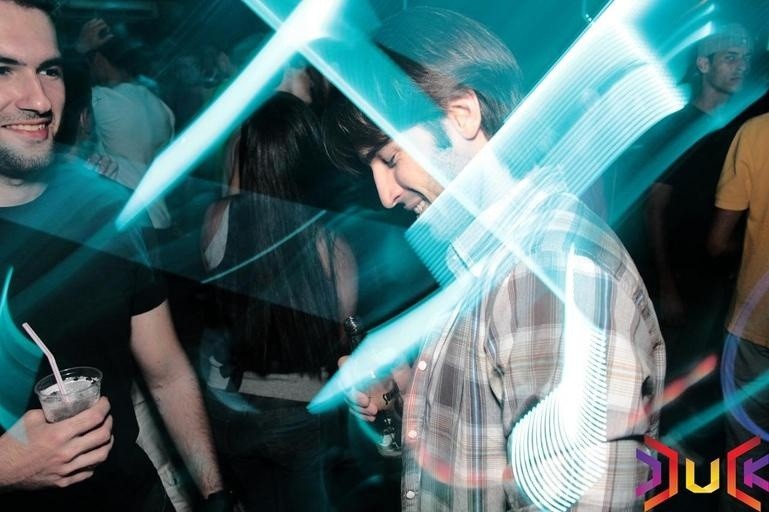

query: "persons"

left=2, top=2, right=769, bottom=511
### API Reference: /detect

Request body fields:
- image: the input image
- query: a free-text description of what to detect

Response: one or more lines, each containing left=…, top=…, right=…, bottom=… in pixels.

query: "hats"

left=675, top=23, right=751, bottom=85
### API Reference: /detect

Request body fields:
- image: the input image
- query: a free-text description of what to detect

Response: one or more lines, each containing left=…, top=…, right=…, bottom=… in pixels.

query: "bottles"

left=341, top=312, right=405, bottom=458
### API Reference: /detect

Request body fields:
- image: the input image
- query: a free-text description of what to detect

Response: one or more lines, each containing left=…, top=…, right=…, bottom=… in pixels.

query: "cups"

left=33, top=364, right=103, bottom=424
